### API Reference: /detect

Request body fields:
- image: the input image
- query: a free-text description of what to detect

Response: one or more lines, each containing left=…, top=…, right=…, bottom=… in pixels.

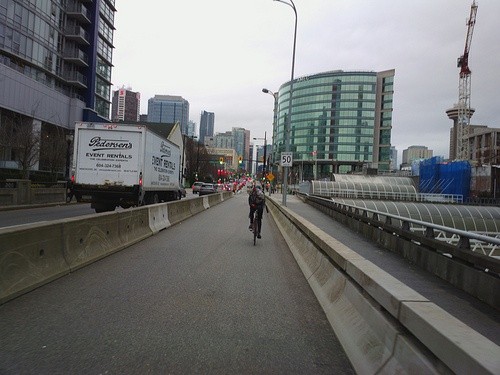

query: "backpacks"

left=248, top=187, right=265, bottom=209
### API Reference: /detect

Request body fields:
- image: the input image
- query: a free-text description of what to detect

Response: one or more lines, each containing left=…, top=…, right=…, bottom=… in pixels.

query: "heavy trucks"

left=67, top=122, right=188, bottom=213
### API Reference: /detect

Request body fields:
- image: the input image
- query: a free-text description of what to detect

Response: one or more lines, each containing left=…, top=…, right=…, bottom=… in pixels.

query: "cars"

left=214, top=179, right=246, bottom=193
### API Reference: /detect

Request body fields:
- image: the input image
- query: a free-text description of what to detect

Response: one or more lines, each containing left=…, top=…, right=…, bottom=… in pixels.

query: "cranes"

left=456, top=0, right=479, bottom=158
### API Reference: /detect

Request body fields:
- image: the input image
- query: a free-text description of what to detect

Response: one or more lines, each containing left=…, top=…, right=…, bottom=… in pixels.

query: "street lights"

left=262, top=88, right=278, bottom=194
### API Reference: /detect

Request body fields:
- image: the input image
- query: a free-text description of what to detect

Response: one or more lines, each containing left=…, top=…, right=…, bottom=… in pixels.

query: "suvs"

left=198, top=183, right=215, bottom=197
left=192, top=182, right=204, bottom=194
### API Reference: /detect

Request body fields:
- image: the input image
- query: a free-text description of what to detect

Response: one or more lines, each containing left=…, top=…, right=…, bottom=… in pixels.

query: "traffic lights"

left=239, top=156, right=242, bottom=164
left=220, top=157, right=223, bottom=164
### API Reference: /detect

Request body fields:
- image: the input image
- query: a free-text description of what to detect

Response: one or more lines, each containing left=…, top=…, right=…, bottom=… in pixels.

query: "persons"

left=248, top=186, right=265, bottom=238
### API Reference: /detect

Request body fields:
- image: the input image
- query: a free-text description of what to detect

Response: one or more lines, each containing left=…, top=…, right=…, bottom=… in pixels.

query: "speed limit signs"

left=281, top=152, right=293, bottom=167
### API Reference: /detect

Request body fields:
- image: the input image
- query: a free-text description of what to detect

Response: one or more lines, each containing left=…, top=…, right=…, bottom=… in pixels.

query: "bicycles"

left=248, top=199, right=266, bottom=246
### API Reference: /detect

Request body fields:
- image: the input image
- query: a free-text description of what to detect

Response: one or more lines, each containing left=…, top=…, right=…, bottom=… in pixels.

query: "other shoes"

left=257, top=233, right=262, bottom=239
left=249, top=225, right=253, bottom=230
